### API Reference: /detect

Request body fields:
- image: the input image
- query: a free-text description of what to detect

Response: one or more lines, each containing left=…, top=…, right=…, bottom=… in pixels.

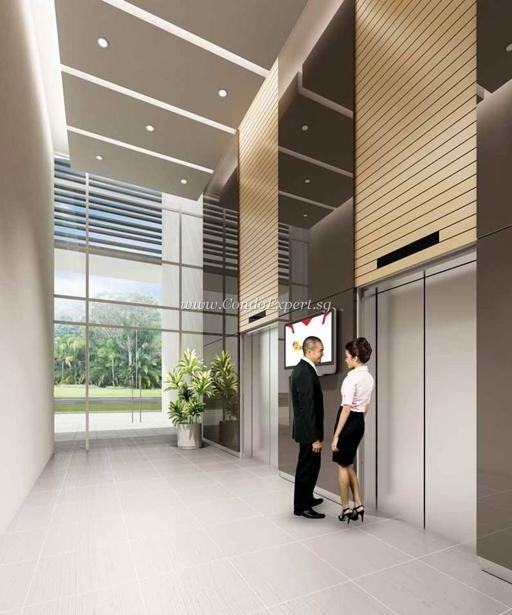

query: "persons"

left=286, top=335, right=327, bottom=520
left=330, top=336, right=374, bottom=525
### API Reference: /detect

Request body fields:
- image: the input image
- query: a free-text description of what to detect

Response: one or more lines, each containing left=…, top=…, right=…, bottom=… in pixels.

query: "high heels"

left=351, top=504, right=365, bottom=523
left=338, top=508, right=353, bottom=525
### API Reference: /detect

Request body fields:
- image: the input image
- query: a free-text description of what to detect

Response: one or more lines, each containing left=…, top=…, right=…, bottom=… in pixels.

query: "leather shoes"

left=310, top=498, right=323, bottom=508
left=294, top=509, right=326, bottom=519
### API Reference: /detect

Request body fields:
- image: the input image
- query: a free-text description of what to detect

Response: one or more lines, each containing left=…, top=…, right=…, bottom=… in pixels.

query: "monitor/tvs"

left=284, top=308, right=336, bottom=377
